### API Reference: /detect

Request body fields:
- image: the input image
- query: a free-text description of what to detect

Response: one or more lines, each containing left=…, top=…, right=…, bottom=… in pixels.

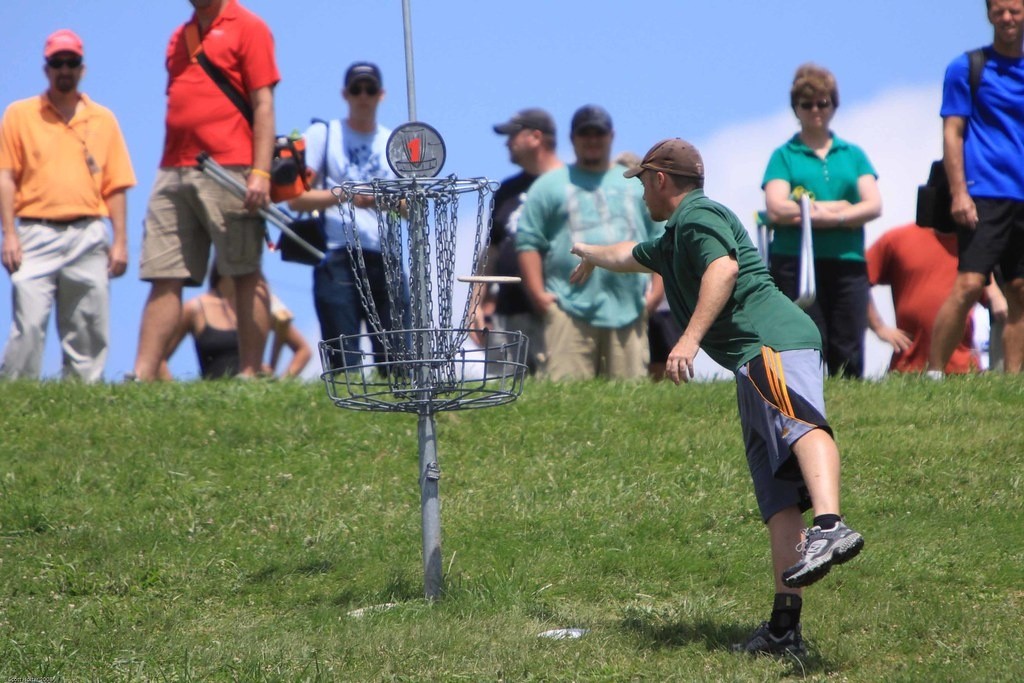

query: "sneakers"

left=730, top=623, right=805, bottom=657
left=782, top=520, right=864, bottom=588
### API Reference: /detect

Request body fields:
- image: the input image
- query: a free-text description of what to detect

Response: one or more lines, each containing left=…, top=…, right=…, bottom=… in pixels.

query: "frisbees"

left=457, top=275, right=522, bottom=283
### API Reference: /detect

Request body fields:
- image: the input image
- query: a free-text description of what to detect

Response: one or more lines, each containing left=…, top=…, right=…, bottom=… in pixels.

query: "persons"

left=865, top=226, right=1008, bottom=380
left=464, top=105, right=569, bottom=384
left=134, top=0, right=282, bottom=381
left=571, top=134, right=866, bottom=659
left=287, top=60, right=408, bottom=379
left=157, top=243, right=312, bottom=389
left=759, top=62, right=882, bottom=382
left=923, top=1, right=1024, bottom=380
left=647, top=272, right=687, bottom=383
left=512, top=105, right=667, bottom=386
left=0, top=28, right=138, bottom=389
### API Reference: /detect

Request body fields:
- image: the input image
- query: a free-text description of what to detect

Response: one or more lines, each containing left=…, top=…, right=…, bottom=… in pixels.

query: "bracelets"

left=251, top=167, right=273, bottom=180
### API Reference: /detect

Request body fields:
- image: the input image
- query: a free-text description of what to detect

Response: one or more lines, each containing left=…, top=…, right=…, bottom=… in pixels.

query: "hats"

left=572, top=106, right=612, bottom=134
left=493, top=108, right=556, bottom=133
left=44, top=30, right=82, bottom=58
left=623, top=137, right=704, bottom=179
left=345, top=63, right=379, bottom=87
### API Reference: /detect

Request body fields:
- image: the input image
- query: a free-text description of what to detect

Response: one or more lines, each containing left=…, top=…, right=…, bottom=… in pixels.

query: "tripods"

left=191, top=147, right=329, bottom=264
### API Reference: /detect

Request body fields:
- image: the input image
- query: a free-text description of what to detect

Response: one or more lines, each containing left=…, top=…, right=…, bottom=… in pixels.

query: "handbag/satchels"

left=917, top=160, right=960, bottom=230
left=276, top=217, right=326, bottom=265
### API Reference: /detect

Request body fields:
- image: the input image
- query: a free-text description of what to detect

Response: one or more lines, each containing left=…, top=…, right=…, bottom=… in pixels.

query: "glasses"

left=348, top=86, right=377, bottom=96
left=797, top=101, right=832, bottom=109
left=47, top=58, right=81, bottom=68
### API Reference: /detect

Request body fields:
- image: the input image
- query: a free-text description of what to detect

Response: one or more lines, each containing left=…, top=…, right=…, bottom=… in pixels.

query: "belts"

left=20, top=216, right=86, bottom=225
